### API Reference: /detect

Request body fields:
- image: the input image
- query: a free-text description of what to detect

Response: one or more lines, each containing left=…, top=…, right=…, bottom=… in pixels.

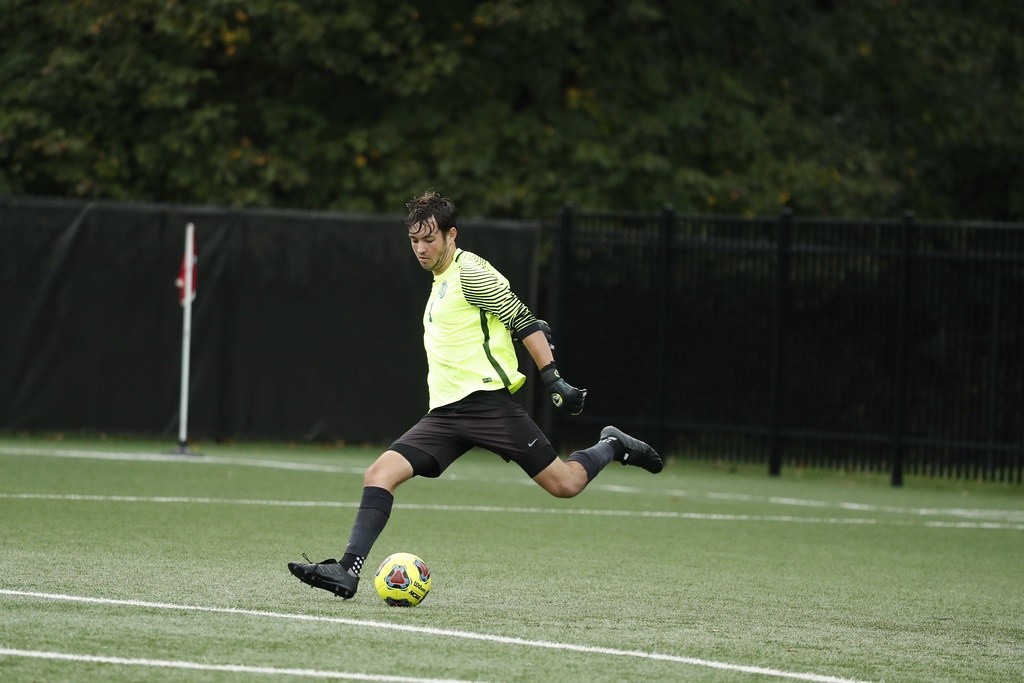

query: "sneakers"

left=287, top=553, right=360, bottom=600
left=600, top=426, right=663, bottom=474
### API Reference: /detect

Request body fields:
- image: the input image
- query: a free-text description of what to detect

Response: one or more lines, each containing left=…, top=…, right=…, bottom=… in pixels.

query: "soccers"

left=376, top=552, right=433, bottom=607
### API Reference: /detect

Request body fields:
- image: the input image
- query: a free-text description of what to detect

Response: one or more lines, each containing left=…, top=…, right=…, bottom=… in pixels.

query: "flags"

left=175, top=231, right=197, bottom=304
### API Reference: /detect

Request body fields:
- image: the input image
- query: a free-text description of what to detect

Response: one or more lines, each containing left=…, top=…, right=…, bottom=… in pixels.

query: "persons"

left=287, top=192, right=664, bottom=600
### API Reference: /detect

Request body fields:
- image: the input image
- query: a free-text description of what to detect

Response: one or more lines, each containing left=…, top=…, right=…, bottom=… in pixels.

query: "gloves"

left=539, top=361, right=588, bottom=416
